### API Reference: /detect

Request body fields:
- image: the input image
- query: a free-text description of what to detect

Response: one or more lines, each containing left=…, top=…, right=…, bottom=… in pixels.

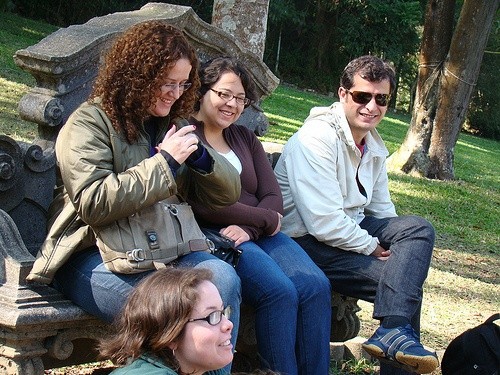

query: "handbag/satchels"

left=92, top=201, right=215, bottom=275
left=201, top=225, right=243, bottom=269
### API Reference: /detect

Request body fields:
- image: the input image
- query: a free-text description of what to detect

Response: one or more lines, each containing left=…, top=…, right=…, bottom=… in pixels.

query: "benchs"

left=0, top=2, right=362, bottom=375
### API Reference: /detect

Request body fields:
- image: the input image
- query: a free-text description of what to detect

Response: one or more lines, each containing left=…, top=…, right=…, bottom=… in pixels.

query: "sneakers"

left=361, top=323, right=440, bottom=373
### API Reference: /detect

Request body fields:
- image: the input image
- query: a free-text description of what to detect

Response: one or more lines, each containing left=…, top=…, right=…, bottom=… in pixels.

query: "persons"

left=99, top=267, right=234, bottom=375
left=274, top=56, right=440, bottom=375
left=26, top=21, right=242, bottom=375
left=178, top=57, right=332, bottom=375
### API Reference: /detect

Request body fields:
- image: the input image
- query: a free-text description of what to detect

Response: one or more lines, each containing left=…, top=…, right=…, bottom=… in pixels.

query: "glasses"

left=158, top=83, right=193, bottom=92
left=209, top=88, right=251, bottom=106
left=347, top=90, right=392, bottom=107
left=185, top=304, right=232, bottom=326
